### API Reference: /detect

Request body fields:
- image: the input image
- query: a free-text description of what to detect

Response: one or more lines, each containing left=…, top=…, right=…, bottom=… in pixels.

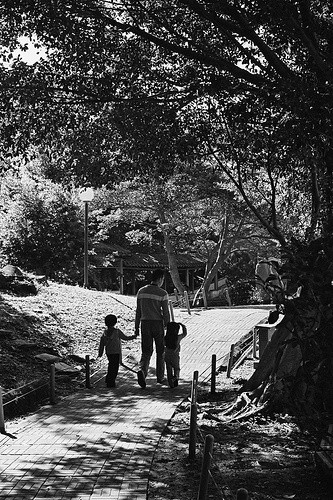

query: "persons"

left=162, top=321, right=188, bottom=388
left=97, top=314, right=137, bottom=388
left=134, top=269, right=171, bottom=389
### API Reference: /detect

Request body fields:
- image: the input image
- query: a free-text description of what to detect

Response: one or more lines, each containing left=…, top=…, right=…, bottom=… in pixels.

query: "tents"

left=91, top=241, right=204, bottom=296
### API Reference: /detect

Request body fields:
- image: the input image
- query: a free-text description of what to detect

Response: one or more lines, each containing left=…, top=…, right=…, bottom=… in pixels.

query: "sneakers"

left=168, top=376, right=174, bottom=388
left=173, top=376, right=178, bottom=387
left=137, top=369, right=146, bottom=390
left=104, top=380, right=115, bottom=388
